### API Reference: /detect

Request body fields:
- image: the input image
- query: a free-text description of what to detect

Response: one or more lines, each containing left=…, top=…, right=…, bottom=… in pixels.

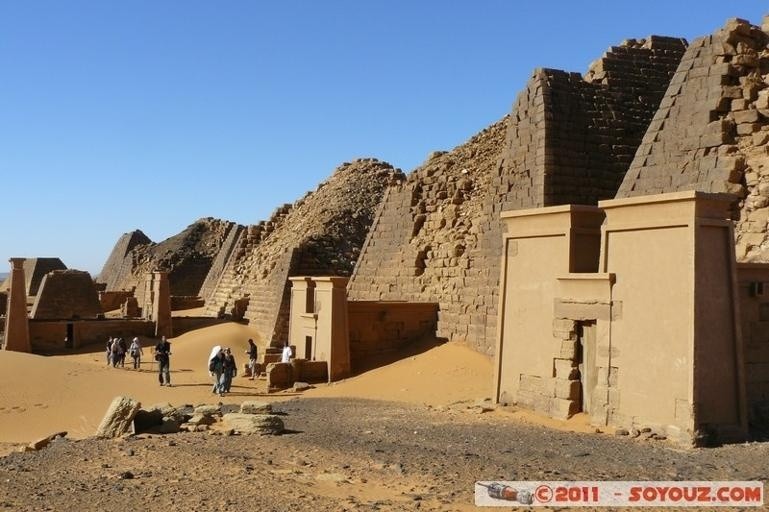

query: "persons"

left=106, top=336, right=114, bottom=365
left=244, top=339, right=259, bottom=380
left=154, top=335, right=172, bottom=387
left=118, top=338, right=127, bottom=368
left=281, top=341, right=292, bottom=362
left=208, top=346, right=225, bottom=397
left=110, top=337, right=123, bottom=367
left=128, top=337, right=144, bottom=371
left=221, top=348, right=238, bottom=392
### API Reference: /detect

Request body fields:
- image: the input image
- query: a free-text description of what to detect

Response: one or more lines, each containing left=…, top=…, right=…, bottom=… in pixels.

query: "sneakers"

left=211, top=388, right=231, bottom=397
left=160, top=383, right=172, bottom=387
